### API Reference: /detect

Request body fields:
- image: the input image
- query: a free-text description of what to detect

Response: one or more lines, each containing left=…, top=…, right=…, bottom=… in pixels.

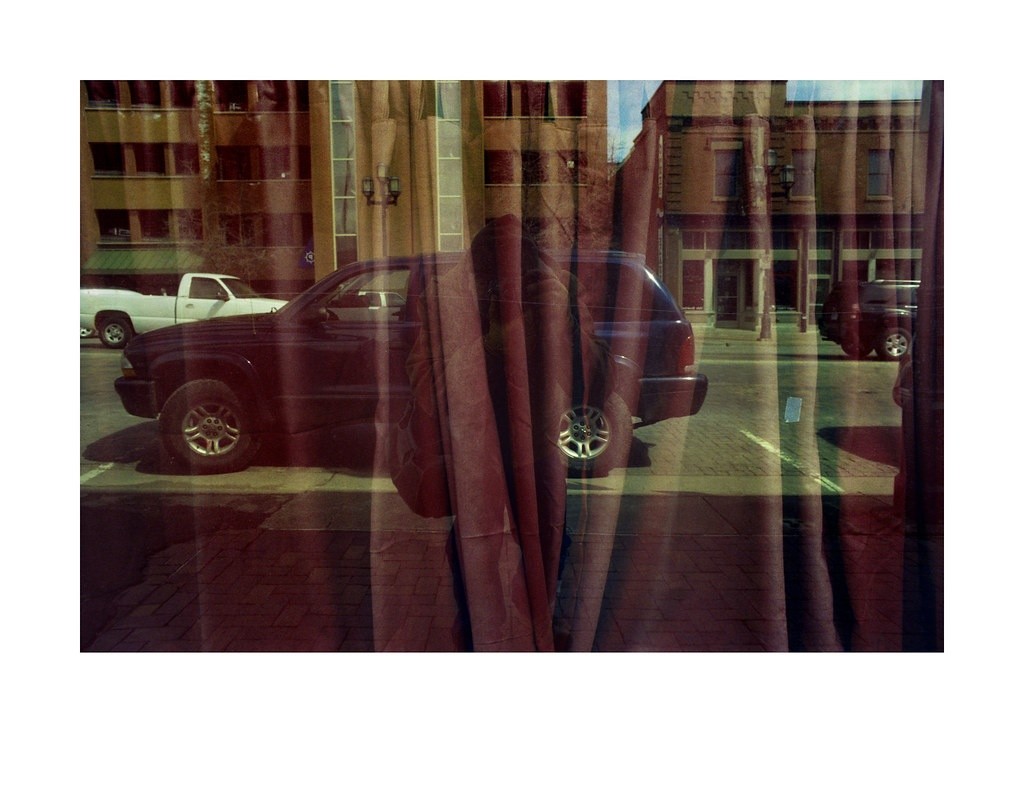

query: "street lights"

left=360, top=161, right=402, bottom=258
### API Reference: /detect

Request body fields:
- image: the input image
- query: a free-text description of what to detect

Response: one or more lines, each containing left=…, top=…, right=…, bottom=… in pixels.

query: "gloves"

left=482, top=294, right=538, bottom=360
left=524, top=278, right=579, bottom=336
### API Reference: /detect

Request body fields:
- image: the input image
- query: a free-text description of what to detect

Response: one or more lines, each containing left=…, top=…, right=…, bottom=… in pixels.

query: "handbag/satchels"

left=390, top=397, right=453, bottom=519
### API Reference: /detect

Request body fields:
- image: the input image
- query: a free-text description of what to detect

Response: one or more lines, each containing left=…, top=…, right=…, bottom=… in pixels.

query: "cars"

left=354, top=290, right=408, bottom=319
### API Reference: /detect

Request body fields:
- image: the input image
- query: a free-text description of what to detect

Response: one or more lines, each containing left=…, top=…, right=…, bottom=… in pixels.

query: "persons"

left=389, top=213, right=618, bottom=652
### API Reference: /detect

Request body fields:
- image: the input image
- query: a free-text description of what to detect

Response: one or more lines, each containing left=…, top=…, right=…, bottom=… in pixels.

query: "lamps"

left=766, top=148, right=796, bottom=203
left=360, top=162, right=402, bottom=207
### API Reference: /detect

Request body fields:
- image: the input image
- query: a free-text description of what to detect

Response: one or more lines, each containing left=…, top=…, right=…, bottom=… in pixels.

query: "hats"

left=467, top=214, right=540, bottom=284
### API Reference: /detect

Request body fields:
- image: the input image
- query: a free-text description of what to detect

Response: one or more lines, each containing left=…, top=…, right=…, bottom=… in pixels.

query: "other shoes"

left=552, top=624, right=573, bottom=652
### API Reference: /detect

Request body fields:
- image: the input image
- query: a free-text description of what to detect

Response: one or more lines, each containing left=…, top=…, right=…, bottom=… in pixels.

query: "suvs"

left=816, top=279, right=921, bottom=360
left=111, top=250, right=710, bottom=478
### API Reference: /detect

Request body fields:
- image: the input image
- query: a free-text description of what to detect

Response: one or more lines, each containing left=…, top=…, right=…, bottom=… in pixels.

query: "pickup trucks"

left=79, top=272, right=297, bottom=350
